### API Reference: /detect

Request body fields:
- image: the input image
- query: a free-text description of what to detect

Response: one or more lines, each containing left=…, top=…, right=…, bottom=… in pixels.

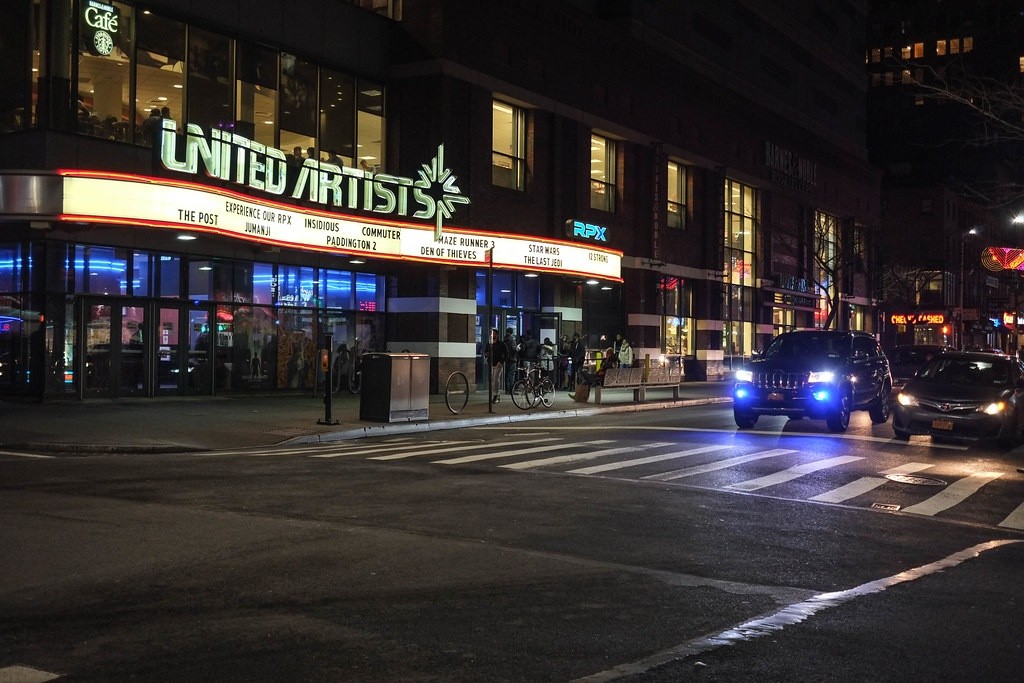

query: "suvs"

left=733, top=331, right=893, bottom=432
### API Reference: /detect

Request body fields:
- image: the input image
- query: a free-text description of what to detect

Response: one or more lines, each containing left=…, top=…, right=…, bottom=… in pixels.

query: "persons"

left=504, top=328, right=555, bottom=395
left=294, top=147, right=305, bottom=166
left=558, top=333, right=586, bottom=391
left=69, top=98, right=89, bottom=132
left=142, top=107, right=172, bottom=145
left=568, top=347, right=619, bottom=400
left=307, top=147, right=314, bottom=159
left=321, top=150, right=343, bottom=169
left=360, top=160, right=377, bottom=175
left=485, top=330, right=509, bottom=404
left=112, top=117, right=121, bottom=139
left=129, top=322, right=144, bottom=392
left=614, top=334, right=633, bottom=369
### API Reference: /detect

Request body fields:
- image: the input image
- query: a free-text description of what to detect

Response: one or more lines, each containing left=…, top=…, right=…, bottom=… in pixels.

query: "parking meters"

left=321, top=335, right=332, bottom=420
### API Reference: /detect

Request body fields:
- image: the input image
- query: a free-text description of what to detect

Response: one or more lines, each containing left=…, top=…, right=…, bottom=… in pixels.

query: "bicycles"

left=511, top=366, right=556, bottom=409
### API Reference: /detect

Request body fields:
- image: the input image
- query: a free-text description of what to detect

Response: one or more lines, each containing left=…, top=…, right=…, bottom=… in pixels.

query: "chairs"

left=588, top=366, right=682, bottom=404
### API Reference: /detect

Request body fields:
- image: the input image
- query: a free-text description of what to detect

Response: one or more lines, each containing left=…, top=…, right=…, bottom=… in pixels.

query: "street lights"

left=960, top=227, right=979, bottom=351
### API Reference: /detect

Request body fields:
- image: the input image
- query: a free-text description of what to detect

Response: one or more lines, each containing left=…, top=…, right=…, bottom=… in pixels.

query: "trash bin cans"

left=359, top=351, right=432, bottom=423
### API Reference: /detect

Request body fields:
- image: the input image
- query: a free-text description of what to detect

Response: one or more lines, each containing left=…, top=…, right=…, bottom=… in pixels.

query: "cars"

left=893, top=352, right=1023, bottom=448
left=890, top=344, right=1006, bottom=402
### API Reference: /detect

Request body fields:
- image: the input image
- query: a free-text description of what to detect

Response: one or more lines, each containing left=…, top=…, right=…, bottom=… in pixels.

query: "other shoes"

left=492, top=395, right=501, bottom=404
left=568, top=393, right=576, bottom=400
